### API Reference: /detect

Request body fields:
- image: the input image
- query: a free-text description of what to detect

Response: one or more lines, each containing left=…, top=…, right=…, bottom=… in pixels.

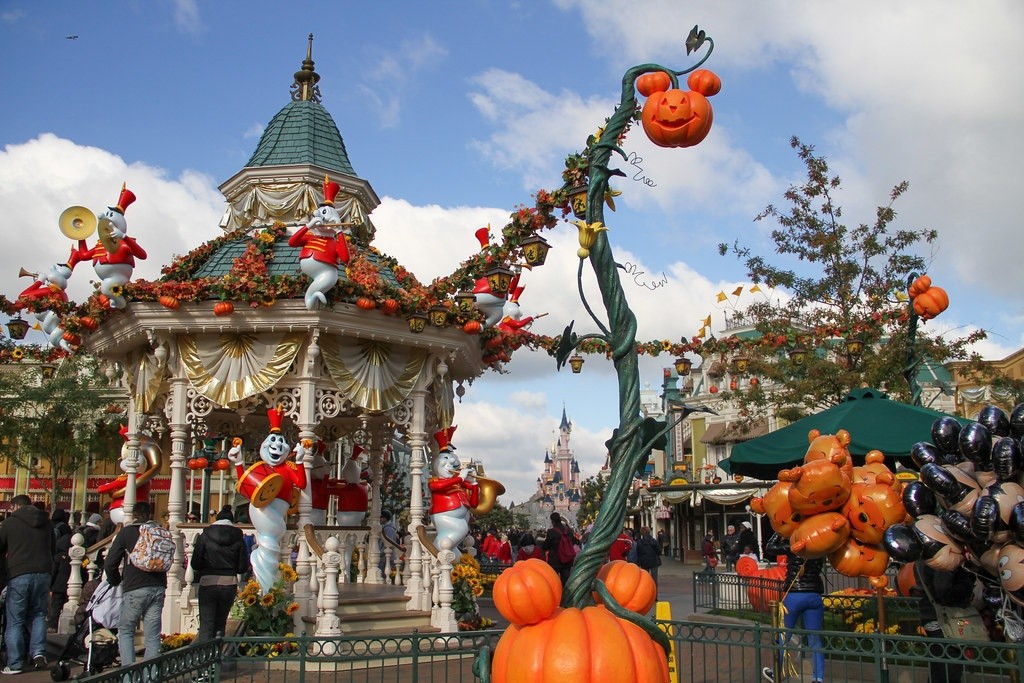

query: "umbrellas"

left=717, top=387, right=979, bottom=481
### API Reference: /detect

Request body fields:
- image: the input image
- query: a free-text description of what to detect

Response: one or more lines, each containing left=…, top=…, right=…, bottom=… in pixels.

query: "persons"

left=695, top=521, right=760, bottom=584
left=0, top=503, right=259, bottom=656
left=103, top=501, right=168, bottom=683
left=379, top=509, right=398, bottom=583
left=74, top=578, right=102, bottom=632
left=457, top=512, right=664, bottom=603
left=190, top=504, right=248, bottom=683
left=762, top=531, right=826, bottom=683
left=0, top=494, right=57, bottom=675
left=913, top=559, right=977, bottom=683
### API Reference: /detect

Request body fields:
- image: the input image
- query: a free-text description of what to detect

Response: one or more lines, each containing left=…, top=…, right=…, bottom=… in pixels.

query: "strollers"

left=48, top=546, right=122, bottom=683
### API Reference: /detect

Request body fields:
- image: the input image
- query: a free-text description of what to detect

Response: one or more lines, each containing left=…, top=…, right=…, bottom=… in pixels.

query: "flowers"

left=822, top=588, right=901, bottom=635
left=159, top=633, right=198, bottom=653
left=451, top=554, right=499, bottom=632
left=232, top=562, right=302, bottom=658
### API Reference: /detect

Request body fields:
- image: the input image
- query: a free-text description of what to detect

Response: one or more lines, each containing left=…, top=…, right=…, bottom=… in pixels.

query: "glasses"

left=188, top=517, right=198, bottom=520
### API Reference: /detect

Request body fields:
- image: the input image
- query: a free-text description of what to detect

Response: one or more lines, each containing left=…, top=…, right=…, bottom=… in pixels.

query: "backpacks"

left=551, top=526, right=577, bottom=564
left=384, top=525, right=403, bottom=547
left=124, top=523, right=176, bottom=572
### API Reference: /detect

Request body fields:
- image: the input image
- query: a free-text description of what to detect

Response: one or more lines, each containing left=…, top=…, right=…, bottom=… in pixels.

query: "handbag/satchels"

left=778, top=602, right=788, bottom=628
left=710, top=557, right=718, bottom=568
left=933, top=602, right=990, bottom=645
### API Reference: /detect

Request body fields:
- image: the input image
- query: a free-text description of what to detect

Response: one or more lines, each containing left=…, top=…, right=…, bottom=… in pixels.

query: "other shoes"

left=695, top=575, right=698, bottom=583
left=47, top=628, right=57, bottom=633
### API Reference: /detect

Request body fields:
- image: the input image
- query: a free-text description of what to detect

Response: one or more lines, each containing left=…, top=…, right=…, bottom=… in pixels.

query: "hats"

left=216, top=505, right=234, bottom=522
left=381, top=509, right=392, bottom=520
left=89, top=513, right=103, bottom=524
left=742, top=522, right=754, bottom=533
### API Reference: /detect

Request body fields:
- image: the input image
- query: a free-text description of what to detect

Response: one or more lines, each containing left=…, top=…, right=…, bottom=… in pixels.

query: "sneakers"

left=190, top=677, right=209, bottom=683
left=198, top=668, right=214, bottom=679
left=0, top=666, right=22, bottom=674
left=763, top=666, right=774, bottom=683
left=33, top=655, right=47, bottom=671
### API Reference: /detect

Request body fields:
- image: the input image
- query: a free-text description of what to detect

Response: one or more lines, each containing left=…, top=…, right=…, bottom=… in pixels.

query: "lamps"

left=406, top=310, right=429, bottom=333
left=565, top=182, right=589, bottom=220
left=845, top=335, right=863, bottom=356
left=789, top=337, right=808, bottom=367
left=569, top=352, right=585, bottom=373
left=40, top=361, right=56, bottom=379
left=673, top=353, right=694, bottom=375
left=483, top=263, right=515, bottom=294
left=454, top=286, right=477, bottom=318
left=427, top=301, right=448, bottom=328
left=519, top=229, right=553, bottom=266
left=5, top=311, right=31, bottom=340
left=731, top=349, right=750, bottom=372
